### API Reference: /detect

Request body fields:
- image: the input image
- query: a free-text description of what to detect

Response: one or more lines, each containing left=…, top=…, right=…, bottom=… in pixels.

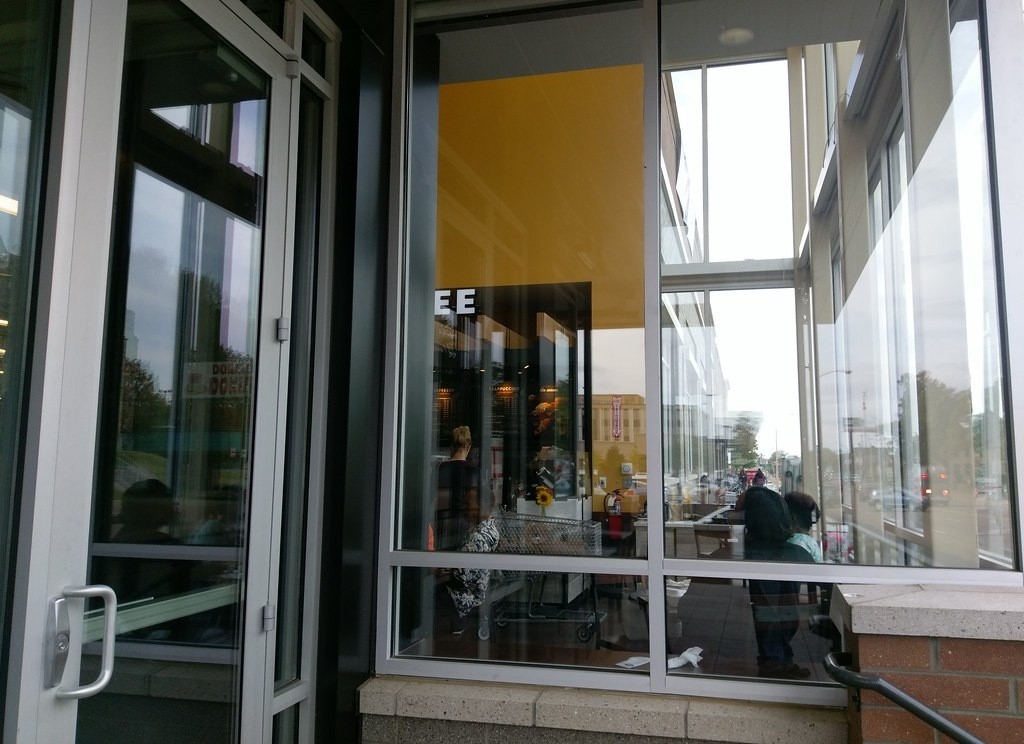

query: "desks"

left=634, top=519, right=746, bottom=588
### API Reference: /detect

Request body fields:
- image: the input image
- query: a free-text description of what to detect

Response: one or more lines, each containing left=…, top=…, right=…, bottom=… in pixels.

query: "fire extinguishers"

left=603, top=488, right=624, bottom=540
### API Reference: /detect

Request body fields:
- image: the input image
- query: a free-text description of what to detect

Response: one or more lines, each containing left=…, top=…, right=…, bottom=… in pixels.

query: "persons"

left=784, top=491, right=824, bottom=564
left=187, top=483, right=240, bottom=548
left=677, top=485, right=817, bottom=680
left=752, top=468, right=766, bottom=487
left=97, top=479, right=225, bottom=639
left=738, top=469, right=747, bottom=490
left=426, top=485, right=500, bottom=635
left=439, top=426, right=481, bottom=483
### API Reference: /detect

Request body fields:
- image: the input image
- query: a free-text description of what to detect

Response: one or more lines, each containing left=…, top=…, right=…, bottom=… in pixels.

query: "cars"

left=867, top=488, right=928, bottom=511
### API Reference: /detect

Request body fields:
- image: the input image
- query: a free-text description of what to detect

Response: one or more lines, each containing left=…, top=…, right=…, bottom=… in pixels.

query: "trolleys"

left=475, top=510, right=610, bottom=643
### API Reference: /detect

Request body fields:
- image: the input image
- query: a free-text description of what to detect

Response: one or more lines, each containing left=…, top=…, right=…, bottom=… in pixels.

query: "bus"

left=909, top=465, right=952, bottom=509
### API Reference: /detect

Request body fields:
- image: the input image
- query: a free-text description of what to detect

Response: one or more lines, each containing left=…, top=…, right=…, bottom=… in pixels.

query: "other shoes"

left=784, top=664, right=811, bottom=679
left=453, top=627, right=463, bottom=634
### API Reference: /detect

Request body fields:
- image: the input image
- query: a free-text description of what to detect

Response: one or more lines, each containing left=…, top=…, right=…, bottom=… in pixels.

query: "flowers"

left=536, top=490, right=552, bottom=516
